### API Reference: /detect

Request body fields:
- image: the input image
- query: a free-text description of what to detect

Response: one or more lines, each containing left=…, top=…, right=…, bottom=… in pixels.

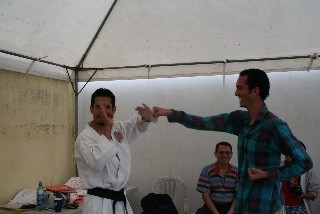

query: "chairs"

left=154, top=176, right=189, bottom=214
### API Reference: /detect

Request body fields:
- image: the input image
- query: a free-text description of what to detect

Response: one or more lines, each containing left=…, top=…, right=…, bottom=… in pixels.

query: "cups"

left=54, top=198, right=63, bottom=212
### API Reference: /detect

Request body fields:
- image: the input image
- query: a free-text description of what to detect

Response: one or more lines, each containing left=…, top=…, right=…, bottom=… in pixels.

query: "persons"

left=280, top=141, right=320, bottom=214
left=195, top=141, right=238, bottom=214
left=73, top=88, right=156, bottom=214
left=152, top=69, right=313, bottom=214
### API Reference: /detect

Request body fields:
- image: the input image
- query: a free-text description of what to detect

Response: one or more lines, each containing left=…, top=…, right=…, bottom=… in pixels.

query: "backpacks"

left=141, top=192, right=179, bottom=214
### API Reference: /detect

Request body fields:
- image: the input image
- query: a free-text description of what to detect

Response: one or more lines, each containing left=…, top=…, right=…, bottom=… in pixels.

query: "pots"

left=44, top=190, right=70, bottom=209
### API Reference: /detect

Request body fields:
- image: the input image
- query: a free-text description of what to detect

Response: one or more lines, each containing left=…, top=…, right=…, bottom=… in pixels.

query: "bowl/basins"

left=74, top=199, right=85, bottom=208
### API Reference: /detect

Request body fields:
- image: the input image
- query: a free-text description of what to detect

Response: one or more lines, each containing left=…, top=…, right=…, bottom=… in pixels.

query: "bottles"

left=36, top=180, right=44, bottom=211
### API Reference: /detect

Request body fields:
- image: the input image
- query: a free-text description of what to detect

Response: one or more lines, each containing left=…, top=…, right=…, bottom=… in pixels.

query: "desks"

left=0, top=186, right=137, bottom=214
